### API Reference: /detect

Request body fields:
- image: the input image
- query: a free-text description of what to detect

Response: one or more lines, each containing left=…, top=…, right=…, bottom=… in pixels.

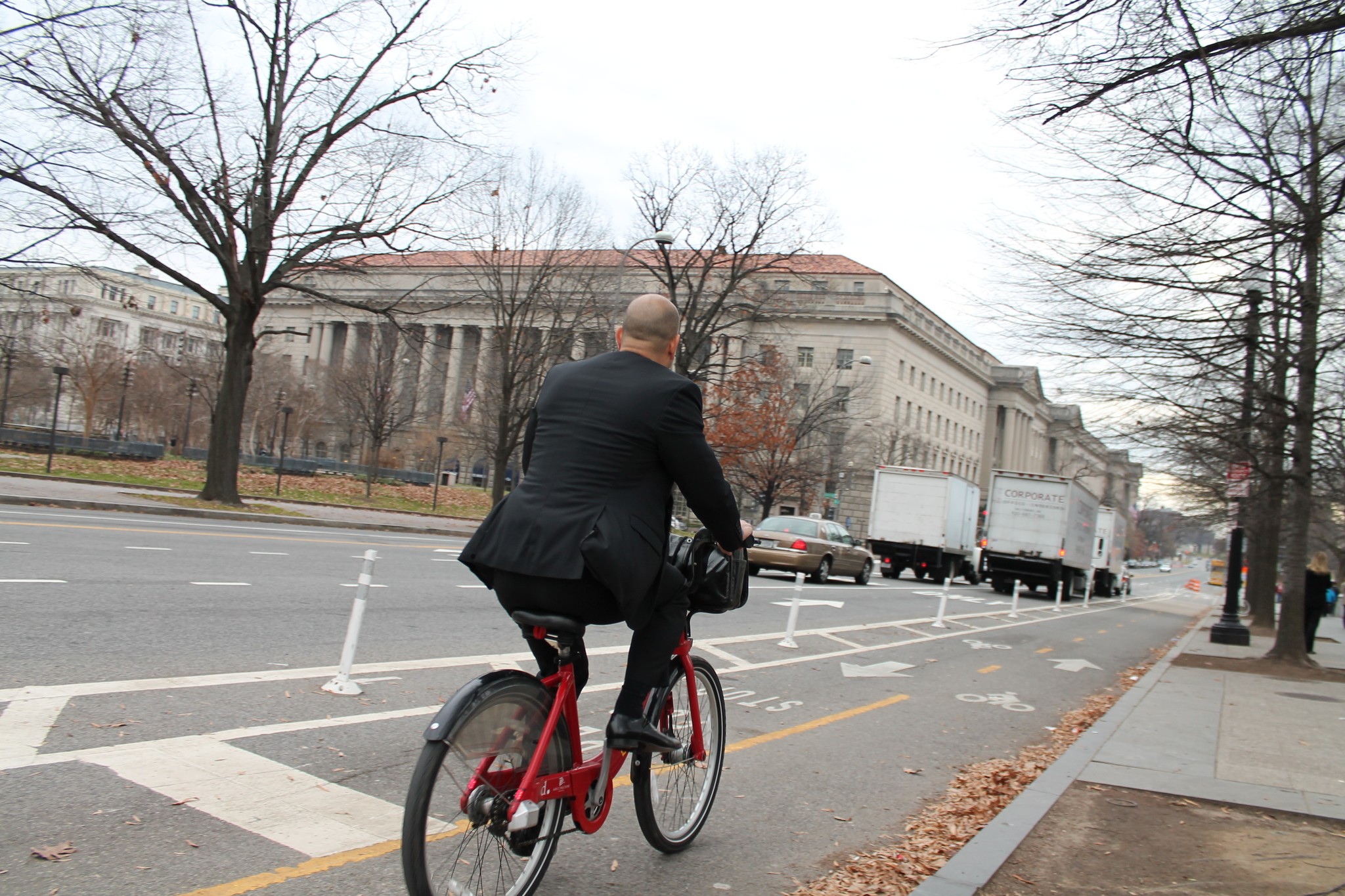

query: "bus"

left=1210, top=561, right=1225, bottom=586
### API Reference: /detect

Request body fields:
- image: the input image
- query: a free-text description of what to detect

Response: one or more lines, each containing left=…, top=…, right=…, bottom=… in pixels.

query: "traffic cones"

left=1186, top=576, right=1202, bottom=591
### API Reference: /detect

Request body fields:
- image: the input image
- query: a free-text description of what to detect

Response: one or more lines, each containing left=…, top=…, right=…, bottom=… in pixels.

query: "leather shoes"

left=509, top=817, right=544, bottom=857
left=606, top=712, right=682, bottom=754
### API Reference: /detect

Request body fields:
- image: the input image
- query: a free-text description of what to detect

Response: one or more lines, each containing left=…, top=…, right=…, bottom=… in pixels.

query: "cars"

left=1126, top=554, right=1209, bottom=573
left=748, top=513, right=873, bottom=585
left=1115, top=567, right=1133, bottom=597
left=671, top=515, right=688, bottom=532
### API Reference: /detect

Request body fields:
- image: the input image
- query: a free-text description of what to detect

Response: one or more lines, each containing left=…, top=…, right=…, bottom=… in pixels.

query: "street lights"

left=606, top=232, right=673, bottom=358
left=825, top=350, right=868, bottom=443
left=267, top=383, right=285, bottom=460
left=362, top=351, right=412, bottom=475
left=866, top=418, right=879, bottom=482
left=115, top=355, right=133, bottom=440
left=181, top=372, right=199, bottom=447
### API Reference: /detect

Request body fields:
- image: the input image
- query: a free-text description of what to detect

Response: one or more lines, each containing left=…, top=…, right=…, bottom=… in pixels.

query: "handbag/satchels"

left=669, top=532, right=750, bottom=614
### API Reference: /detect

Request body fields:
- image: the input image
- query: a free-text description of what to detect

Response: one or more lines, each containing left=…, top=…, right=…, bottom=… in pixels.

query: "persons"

left=1325, top=589, right=1336, bottom=615
left=1275, top=580, right=1283, bottom=603
left=1305, top=550, right=1332, bottom=654
left=456, top=291, right=754, bottom=858
left=1330, top=581, right=1340, bottom=613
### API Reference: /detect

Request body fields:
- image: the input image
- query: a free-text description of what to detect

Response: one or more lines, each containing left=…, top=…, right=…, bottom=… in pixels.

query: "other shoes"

left=1307, top=650, right=1316, bottom=654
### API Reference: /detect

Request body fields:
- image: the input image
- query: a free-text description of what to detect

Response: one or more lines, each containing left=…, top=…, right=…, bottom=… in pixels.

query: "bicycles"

left=401, top=529, right=762, bottom=895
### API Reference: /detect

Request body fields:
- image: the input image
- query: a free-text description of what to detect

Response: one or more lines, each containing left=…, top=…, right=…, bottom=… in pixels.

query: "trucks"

left=1083, top=506, right=1129, bottom=595
left=981, top=467, right=1098, bottom=604
left=869, top=462, right=981, bottom=583
